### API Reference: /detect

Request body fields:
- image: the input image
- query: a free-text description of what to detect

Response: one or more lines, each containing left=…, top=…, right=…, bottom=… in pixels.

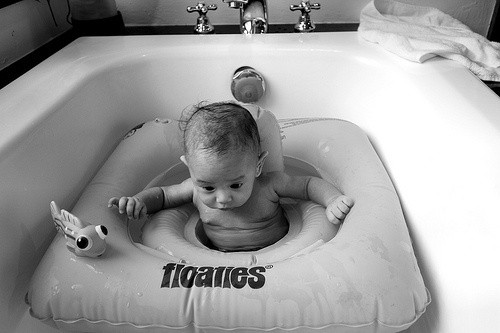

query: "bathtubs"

left=0, top=32, right=500, bottom=333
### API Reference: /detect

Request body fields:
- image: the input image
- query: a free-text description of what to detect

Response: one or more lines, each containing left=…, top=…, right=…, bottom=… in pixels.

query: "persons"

left=107, top=99, right=354, bottom=252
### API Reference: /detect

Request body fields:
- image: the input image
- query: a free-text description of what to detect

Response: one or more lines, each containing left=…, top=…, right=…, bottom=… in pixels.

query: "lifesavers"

left=25, top=116, right=432, bottom=333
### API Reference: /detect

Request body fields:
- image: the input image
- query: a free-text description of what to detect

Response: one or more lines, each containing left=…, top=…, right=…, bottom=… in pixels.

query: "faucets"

left=223, top=0, right=268, bottom=34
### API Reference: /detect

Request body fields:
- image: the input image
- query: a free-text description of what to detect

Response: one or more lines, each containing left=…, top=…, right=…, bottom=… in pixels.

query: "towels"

left=358, top=0, right=500, bottom=81
